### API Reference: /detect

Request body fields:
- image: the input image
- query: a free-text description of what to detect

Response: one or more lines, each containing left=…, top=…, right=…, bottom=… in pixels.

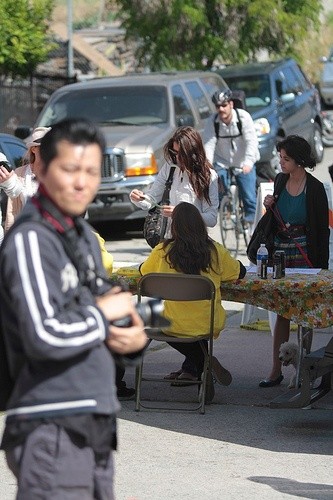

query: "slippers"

left=164, top=371, right=181, bottom=381
left=170, top=375, right=198, bottom=387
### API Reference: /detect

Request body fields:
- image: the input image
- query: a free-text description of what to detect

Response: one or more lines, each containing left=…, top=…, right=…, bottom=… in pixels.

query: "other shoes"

left=242, top=220, right=252, bottom=229
left=116, top=382, right=137, bottom=401
left=198, top=371, right=214, bottom=405
left=224, top=206, right=230, bottom=220
left=207, top=356, right=232, bottom=385
left=258, top=374, right=284, bottom=387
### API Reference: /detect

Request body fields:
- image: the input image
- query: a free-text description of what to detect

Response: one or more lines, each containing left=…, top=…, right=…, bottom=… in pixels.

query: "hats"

left=24, top=127, right=54, bottom=148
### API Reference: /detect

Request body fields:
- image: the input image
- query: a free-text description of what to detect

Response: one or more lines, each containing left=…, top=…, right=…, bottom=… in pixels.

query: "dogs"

left=277, top=340, right=333, bottom=390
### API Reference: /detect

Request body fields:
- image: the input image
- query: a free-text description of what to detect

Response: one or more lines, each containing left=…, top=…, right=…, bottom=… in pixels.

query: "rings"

left=266, top=199, right=268, bottom=203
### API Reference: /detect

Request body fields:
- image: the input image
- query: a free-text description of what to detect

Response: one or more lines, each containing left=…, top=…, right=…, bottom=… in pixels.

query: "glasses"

left=216, top=101, right=230, bottom=108
left=168, top=147, right=182, bottom=156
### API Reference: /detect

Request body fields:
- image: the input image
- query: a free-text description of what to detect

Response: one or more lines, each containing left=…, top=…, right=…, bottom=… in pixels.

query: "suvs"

left=14, top=69, right=232, bottom=234
left=213, top=58, right=325, bottom=182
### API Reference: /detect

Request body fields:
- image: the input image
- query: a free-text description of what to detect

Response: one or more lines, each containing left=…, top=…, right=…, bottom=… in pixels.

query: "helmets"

left=212, top=90, right=231, bottom=105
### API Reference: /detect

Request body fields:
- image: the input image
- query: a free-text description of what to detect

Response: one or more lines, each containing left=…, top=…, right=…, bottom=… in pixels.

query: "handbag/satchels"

left=245, top=174, right=290, bottom=267
left=143, top=166, right=176, bottom=249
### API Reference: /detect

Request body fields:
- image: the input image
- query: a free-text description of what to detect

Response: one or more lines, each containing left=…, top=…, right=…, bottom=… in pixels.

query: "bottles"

left=274, top=252, right=281, bottom=280
left=230, top=182, right=237, bottom=195
left=260, top=258, right=268, bottom=280
left=256, top=243, right=268, bottom=276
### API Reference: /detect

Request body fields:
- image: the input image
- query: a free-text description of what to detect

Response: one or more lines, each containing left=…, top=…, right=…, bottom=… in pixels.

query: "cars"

left=0, top=132, right=29, bottom=170
left=318, top=49, right=333, bottom=148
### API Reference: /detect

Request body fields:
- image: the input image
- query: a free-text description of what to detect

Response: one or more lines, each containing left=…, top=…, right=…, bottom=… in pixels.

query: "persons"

left=211, top=89, right=261, bottom=230
left=258, top=135, right=330, bottom=387
left=138, top=202, right=247, bottom=405
left=129, top=125, right=219, bottom=387
left=0, top=116, right=151, bottom=500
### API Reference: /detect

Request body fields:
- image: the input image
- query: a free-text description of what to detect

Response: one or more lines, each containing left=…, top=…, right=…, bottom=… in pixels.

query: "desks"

left=109, top=265, right=333, bottom=387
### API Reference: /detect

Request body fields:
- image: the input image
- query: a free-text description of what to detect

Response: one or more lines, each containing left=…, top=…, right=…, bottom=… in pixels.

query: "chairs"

left=300, top=335, right=333, bottom=407
left=134, top=273, right=213, bottom=413
left=256, top=80, right=270, bottom=100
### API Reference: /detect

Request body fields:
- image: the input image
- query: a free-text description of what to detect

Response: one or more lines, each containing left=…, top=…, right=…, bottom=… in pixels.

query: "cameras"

left=112, top=298, right=173, bottom=330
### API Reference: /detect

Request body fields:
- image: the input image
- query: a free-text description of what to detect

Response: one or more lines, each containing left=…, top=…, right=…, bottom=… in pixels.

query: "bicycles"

left=214, top=161, right=262, bottom=259
